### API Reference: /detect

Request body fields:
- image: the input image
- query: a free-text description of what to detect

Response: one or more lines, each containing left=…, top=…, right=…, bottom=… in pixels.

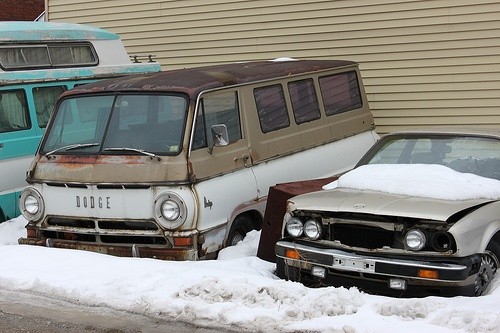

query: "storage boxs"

left=257, top=177, right=344, bottom=263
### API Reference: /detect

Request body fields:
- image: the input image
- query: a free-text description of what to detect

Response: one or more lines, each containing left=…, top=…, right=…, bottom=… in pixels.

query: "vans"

left=13, top=55, right=382, bottom=267
left=0, top=21, right=176, bottom=234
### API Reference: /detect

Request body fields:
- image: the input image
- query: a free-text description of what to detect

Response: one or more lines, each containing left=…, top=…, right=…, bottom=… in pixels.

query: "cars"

left=273, top=129, right=500, bottom=298
